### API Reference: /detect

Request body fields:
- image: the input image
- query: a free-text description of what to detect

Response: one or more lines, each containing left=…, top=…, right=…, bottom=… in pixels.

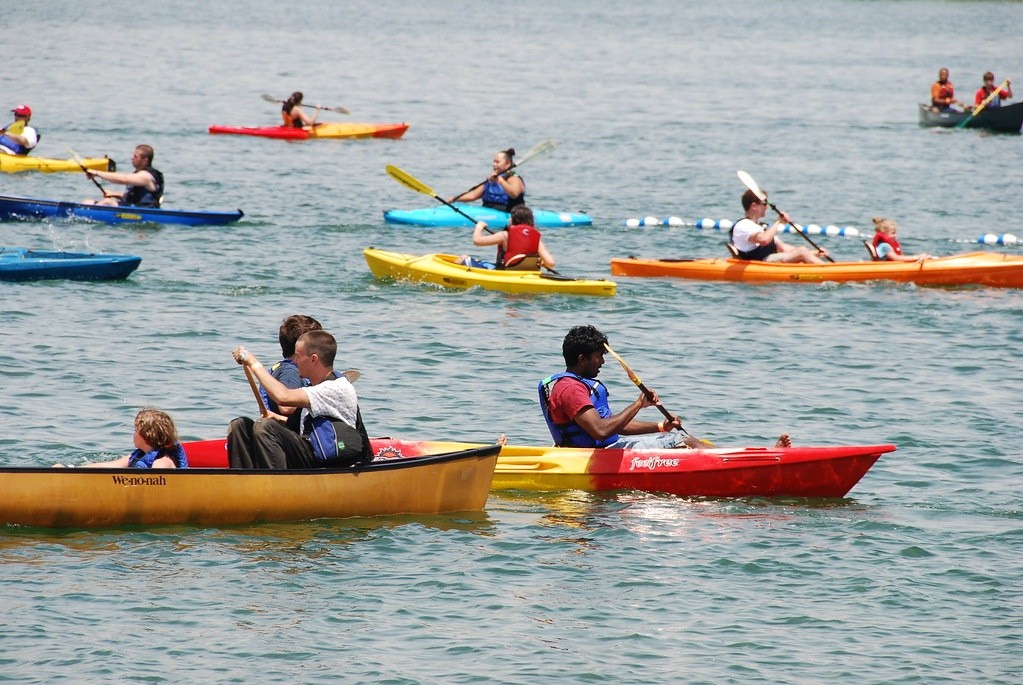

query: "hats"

left=11, top=105, right=31, bottom=121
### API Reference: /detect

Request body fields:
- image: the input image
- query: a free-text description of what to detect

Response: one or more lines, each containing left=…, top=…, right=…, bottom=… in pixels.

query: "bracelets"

left=250, top=361, right=263, bottom=371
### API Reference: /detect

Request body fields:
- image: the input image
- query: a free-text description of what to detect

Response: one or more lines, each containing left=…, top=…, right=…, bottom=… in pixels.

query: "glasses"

left=759, top=203, right=766, bottom=206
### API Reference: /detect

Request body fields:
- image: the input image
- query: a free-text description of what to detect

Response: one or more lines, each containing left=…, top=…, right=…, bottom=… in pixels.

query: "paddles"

left=385, top=164, right=560, bottom=274
left=958, top=80, right=1009, bottom=130
left=64, top=146, right=110, bottom=198
left=737, top=170, right=834, bottom=264
left=0, top=120, right=26, bottom=135
left=604, top=343, right=713, bottom=449
left=449, top=139, right=556, bottom=203
left=259, top=93, right=351, bottom=117
left=238, top=358, right=269, bottom=418
left=912, top=253, right=939, bottom=267
left=342, top=370, right=362, bottom=385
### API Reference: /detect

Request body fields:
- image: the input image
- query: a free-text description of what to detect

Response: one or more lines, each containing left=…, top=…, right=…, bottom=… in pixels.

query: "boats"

left=0, top=438, right=505, bottom=530
left=0, top=193, right=245, bottom=227
left=208, top=120, right=410, bottom=141
left=0, top=244, right=143, bottom=283
left=608, top=250, right=1023, bottom=294
left=0, top=134, right=117, bottom=176
left=129, top=435, right=899, bottom=501
left=916, top=101, right=1023, bottom=135
left=381, top=201, right=594, bottom=230
left=361, top=243, right=621, bottom=301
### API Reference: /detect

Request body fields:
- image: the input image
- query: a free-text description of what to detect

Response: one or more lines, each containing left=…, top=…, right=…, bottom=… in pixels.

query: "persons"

left=972, top=72, right=1012, bottom=113
left=281, top=90, right=321, bottom=128
left=535, top=324, right=794, bottom=448
left=216, top=314, right=373, bottom=471
left=731, top=189, right=830, bottom=264
left=451, top=148, right=526, bottom=213
left=869, top=214, right=938, bottom=261
left=932, top=68, right=958, bottom=115
left=75, top=406, right=189, bottom=469
left=453, top=207, right=557, bottom=274
left=84, top=145, right=166, bottom=209
left=0, top=105, right=41, bottom=155
